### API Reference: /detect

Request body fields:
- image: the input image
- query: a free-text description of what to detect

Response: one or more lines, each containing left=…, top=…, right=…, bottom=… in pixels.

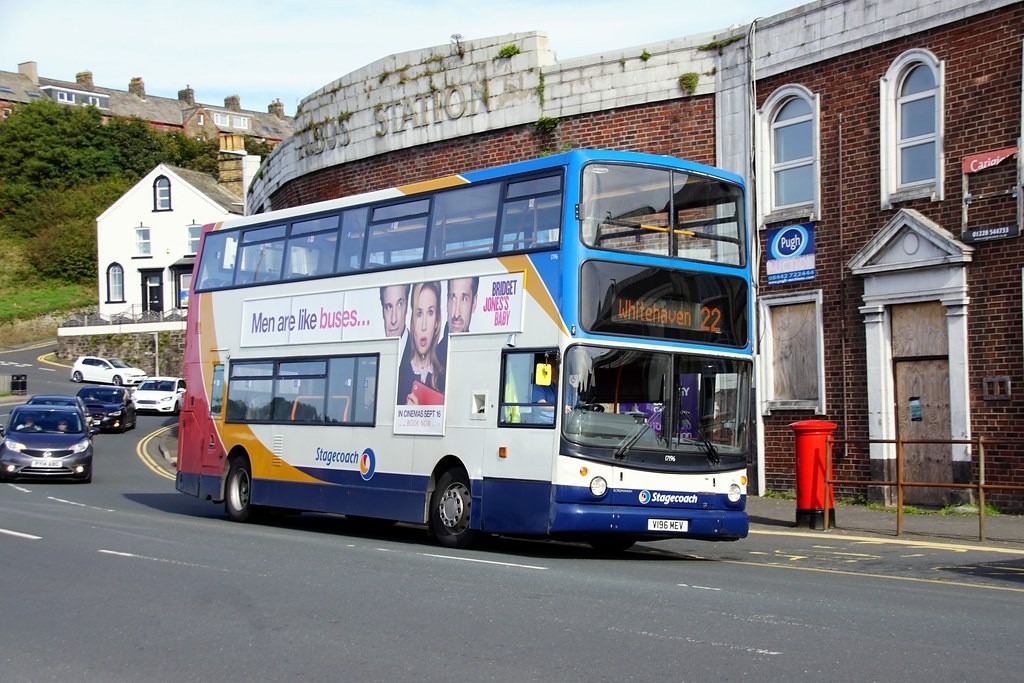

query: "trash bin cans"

left=11, top=374, right=28, bottom=396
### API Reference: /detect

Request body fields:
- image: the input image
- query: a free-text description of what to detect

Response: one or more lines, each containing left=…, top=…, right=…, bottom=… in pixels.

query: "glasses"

left=26, top=418, right=32, bottom=421
left=57, top=423, right=67, bottom=425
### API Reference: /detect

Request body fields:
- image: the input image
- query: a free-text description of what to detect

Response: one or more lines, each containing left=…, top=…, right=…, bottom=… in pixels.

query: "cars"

left=75, top=385, right=137, bottom=435
left=0, top=404, right=100, bottom=483
left=70, top=354, right=149, bottom=388
left=7, top=394, right=95, bottom=432
left=131, top=376, right=187, bottom=417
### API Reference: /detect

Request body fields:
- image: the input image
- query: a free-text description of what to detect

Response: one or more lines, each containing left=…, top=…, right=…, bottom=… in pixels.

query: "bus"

left=171, top=148, right=759, bottom=553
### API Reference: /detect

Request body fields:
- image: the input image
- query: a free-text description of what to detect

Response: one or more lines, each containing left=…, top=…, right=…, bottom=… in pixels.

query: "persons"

left=84, top=391, right=100, bottom=401
left=397, top=281, right=446, bottom=405
left=527, top=355, right=582, bottom=424
left=380, top=283, right=411, bottom=368
left=436, top=276, right=479, bottom=365
left=56, top=418, right=68, bottom=432
left=16, top=414, right=40, bottom=431
